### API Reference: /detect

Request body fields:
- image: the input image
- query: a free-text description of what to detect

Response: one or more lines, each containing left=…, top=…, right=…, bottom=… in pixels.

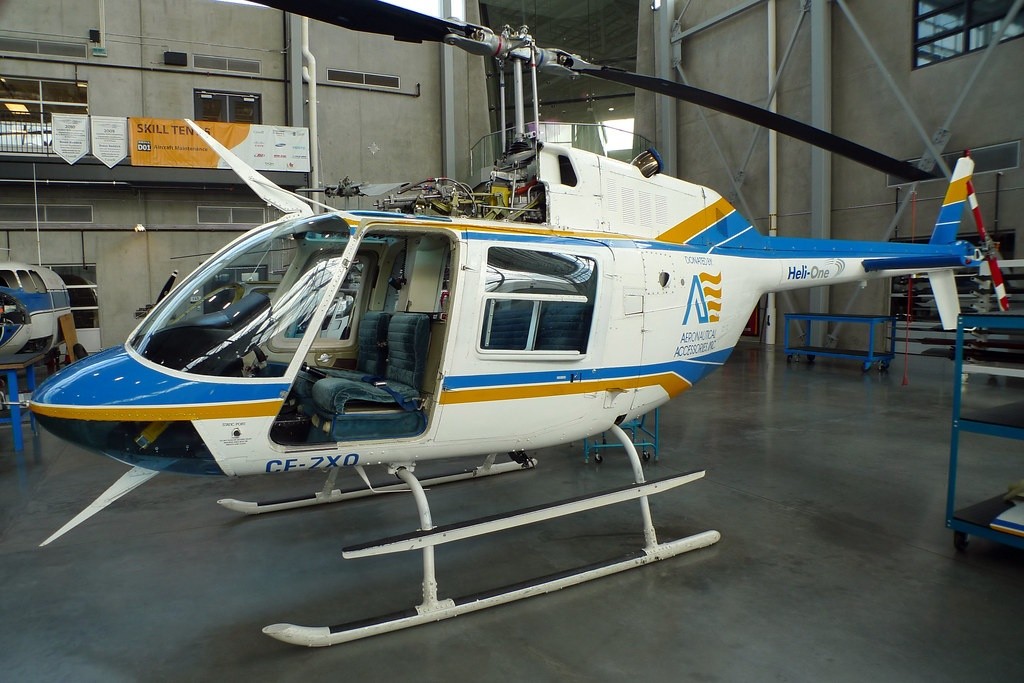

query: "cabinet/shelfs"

left=783, top=313, right=896, bottom=373
left=0, top=353, right=44, bottom=453
left=945, top=313, right=1024, bottom=553
left=570, top=407, right=659, bottom=463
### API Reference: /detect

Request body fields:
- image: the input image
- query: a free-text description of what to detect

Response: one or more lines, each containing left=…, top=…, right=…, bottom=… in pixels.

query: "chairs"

left=533, top=303, right=593, bottom=353
left=292, top=311, right=390, bottom=418
left=312, top=312, right=430, bottom=441
left=480, top=306, right=533, bottom=349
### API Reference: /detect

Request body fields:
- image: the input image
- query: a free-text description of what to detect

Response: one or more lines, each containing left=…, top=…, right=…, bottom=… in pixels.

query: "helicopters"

left=28, top=0, right=1010, bottom=647
left=0, top=248, right=71, bottom=411
